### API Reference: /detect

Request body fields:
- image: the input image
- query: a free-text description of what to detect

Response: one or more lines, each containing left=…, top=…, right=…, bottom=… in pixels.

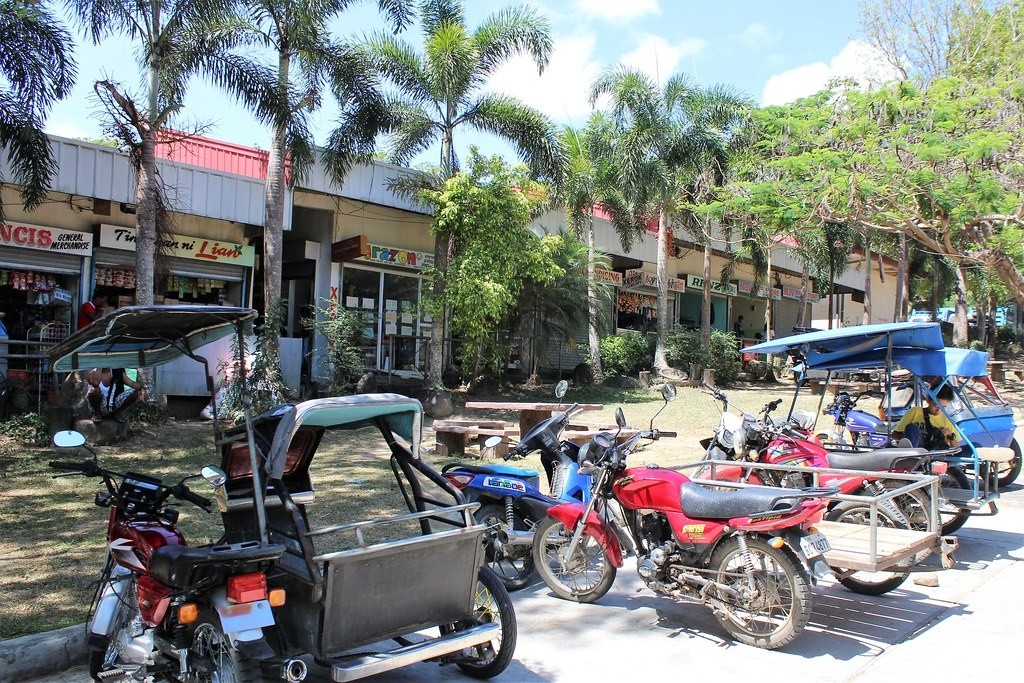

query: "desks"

left=839, top=369, right=884, bottom=392
left=465, top=402, right=604, bottom=449
left=987, top=361, right=1007, bottom=382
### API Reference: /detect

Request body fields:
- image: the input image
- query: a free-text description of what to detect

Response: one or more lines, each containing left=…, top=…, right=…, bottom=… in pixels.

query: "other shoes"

left=114, top=413, right=126, bottom=423
left=92, top=414, right=104, bottom=423
left=898, top=438, right=912, bottom=448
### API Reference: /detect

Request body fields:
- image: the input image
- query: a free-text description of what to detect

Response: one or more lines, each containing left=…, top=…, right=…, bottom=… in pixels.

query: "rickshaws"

left=738, top=322, right=1016, bottom=537
left=218, top=395, right=516, bottom=683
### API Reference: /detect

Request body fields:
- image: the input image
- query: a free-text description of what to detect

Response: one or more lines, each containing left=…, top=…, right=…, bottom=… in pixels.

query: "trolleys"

left=662, top=459, right=958, bottom=595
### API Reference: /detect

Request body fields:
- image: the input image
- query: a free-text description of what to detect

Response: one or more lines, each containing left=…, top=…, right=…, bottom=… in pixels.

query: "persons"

left=734, top=315, right=744, bottom=362
left=78, top=368, right=150, bottom=423
left=892, top=382, right=962, bottom=451
left=762, top=323, right=775, bottom=342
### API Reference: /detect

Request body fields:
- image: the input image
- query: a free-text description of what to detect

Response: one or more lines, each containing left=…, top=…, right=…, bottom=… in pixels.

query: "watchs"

left=947, top=433, right=956, bottom=440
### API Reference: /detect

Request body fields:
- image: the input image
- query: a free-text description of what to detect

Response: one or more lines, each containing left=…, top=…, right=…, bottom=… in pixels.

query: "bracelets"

left=140, top=387, right=146, bottom=390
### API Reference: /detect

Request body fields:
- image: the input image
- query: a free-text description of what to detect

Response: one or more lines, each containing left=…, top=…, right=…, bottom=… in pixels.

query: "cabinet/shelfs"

left=26, top=321, right=70, bottom=416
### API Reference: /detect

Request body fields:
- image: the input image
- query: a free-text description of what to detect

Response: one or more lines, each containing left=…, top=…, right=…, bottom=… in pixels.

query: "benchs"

left=433, top=420, right=514, bottom=444
left=565, top=420, right=632, bottom=431
left=268, top=503, right=487, bottom=652
left=986, top=368, right=992, bottom=380
left=819, top=381, right=879, bottom=401
left=999, top=369, right=1023, bottom=382
left=432, top=426, right=519, bottom=459
left=561, top=430, right=637, bottom=451
left=809, top=380, right=824, bottom=395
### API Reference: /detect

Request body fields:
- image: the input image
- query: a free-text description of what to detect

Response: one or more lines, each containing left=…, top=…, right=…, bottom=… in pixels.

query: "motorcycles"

left=694, top=382, right=819, bottom=479
left=439, top=380, right=634, bottom=591
left=532, top=382, right=842, bottom=651
left=817, top=372, right=1023, bottom=486
left=49, top=304, right=307, bottom=683
left=698, top=400, right=963, bottom=596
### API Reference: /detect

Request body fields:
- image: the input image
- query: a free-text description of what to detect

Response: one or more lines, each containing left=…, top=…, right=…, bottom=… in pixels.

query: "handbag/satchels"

left=922, top=407, right=950, bottom=450
